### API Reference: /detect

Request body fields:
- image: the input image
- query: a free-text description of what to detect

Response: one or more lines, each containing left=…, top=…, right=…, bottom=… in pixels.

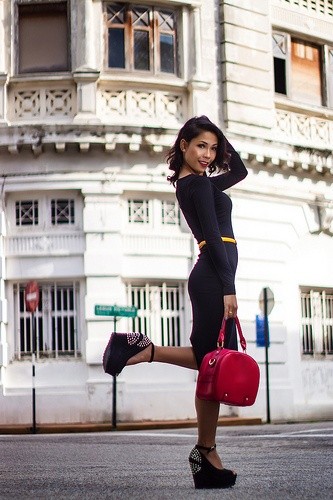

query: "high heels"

left=188, top=445, right=237, bottom=488
left=104, top=332, right=154, bottom=378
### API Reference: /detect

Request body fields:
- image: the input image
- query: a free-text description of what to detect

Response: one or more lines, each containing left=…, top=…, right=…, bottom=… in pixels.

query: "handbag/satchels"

left=197, top=315, right=260, bottom=407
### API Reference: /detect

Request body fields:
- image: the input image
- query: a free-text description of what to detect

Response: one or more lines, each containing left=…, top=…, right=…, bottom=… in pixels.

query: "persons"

left=102, top=115, right=248, bottom=489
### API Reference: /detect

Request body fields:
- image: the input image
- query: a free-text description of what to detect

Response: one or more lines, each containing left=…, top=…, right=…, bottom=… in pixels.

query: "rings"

left=229, top=312, right=233, bottom=315
